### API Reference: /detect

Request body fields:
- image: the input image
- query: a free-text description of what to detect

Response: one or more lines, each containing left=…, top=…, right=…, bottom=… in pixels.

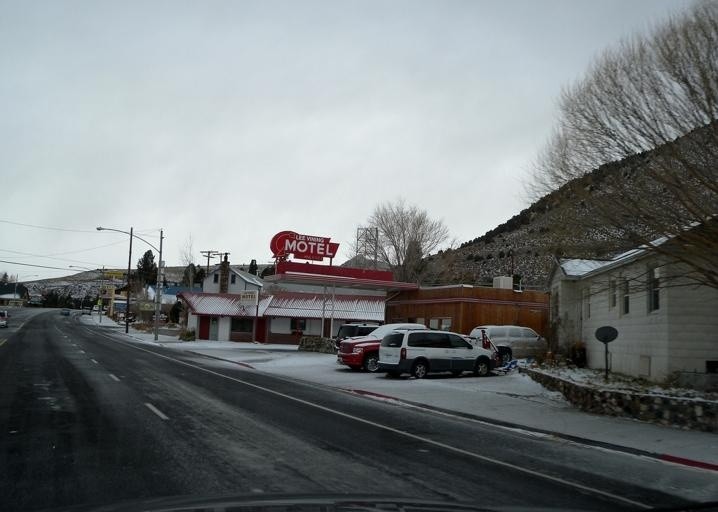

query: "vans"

left=470, top=326, right=548, bottom=367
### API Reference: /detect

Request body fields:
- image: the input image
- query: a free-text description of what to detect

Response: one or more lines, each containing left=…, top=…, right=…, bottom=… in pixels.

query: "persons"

left=299, top=327, right=304, bottom=345
left=294, top=327, right=299, bottom=345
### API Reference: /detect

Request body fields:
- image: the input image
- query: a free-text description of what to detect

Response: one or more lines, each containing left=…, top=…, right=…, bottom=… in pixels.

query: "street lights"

left=69, top=266, right=105, bottom=322
left=97, top=227, right=164, bottom=340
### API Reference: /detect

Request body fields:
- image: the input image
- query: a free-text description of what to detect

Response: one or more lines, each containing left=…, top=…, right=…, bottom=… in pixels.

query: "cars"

left=0, top=310, right=10, bottom=327
left=82, top=305, right=107, bottom=315
left=61, top=308, right=70, bottom=315
left=336, top=323, right=497, bottom=379
